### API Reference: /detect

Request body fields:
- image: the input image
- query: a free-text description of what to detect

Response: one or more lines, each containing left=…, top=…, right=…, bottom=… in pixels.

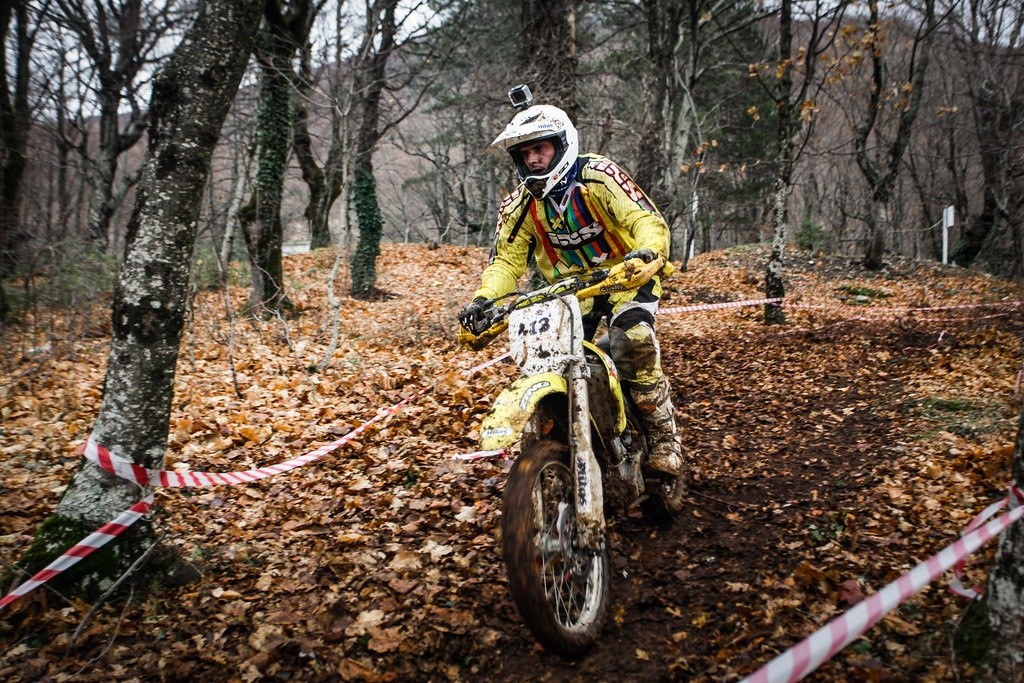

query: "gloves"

left=624, top=248, right=659, bottom=265
left=459, top=296, right=492, bottom=335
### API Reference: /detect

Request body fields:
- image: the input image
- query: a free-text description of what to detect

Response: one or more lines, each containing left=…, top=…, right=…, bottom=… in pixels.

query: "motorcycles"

left=452, top=257, right=686, bottom=659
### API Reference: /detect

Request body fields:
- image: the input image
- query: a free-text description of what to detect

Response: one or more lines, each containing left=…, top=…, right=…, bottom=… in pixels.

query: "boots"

left=630, top=375, right=682, bottom=478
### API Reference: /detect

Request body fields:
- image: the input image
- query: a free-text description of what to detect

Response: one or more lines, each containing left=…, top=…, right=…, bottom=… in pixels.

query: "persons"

left=456, top=104, right=683, bottom=477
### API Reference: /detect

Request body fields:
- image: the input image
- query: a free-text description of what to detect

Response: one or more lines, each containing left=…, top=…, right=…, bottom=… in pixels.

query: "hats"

left=492, top=105, right=579, bottom=203
